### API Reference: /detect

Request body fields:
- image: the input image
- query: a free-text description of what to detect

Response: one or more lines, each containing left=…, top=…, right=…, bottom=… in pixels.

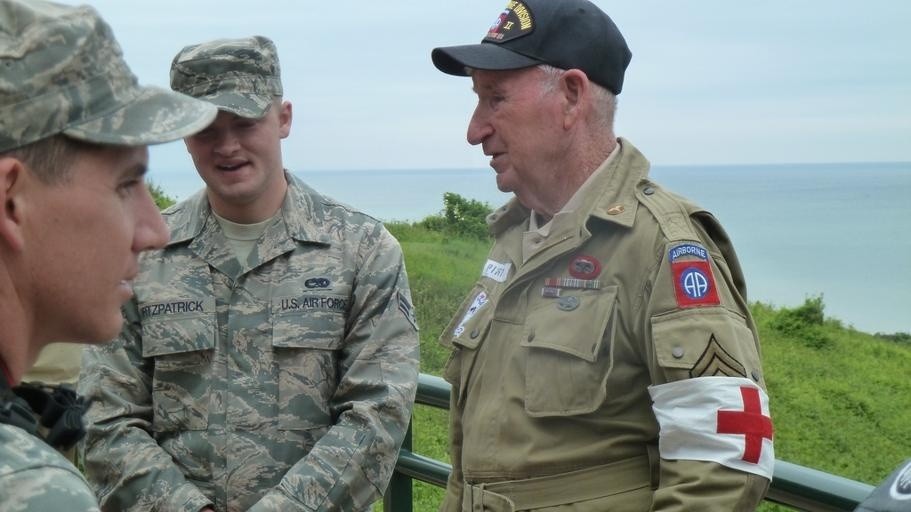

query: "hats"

left=2, top=0, right=219, bottom=155
left=432, top=0, right=632, bottom=95
left=170, top=36, right=283, bottom=118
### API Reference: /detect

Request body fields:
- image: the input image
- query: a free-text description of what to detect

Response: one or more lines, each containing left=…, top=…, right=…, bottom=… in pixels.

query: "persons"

left=429, top=0, right=777, bottom=511
left=0, top=1, right=221, bottom=511
left=76, top=34, right=420, bottom=511
